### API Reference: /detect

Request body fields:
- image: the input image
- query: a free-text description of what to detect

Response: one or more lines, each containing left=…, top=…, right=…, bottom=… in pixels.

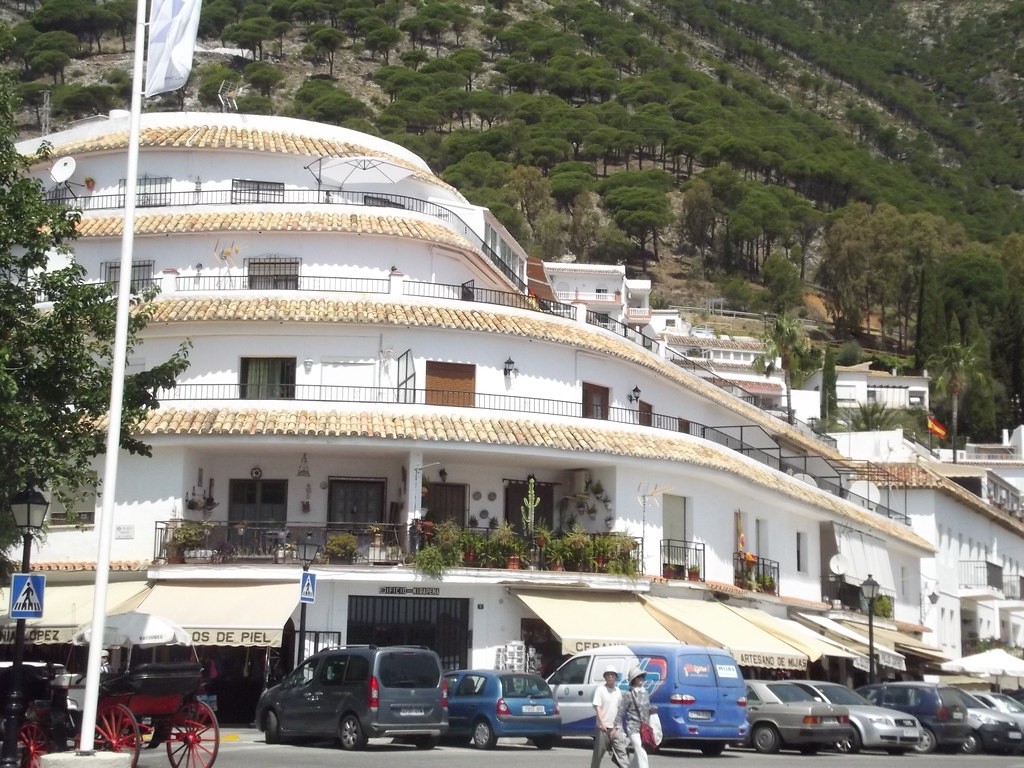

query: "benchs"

left=99, top=660, right=200, bottom=698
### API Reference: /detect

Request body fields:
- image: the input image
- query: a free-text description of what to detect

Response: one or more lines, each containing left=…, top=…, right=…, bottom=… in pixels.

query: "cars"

left=792, top=679, right=924, bottom=755
left=1, top=662, right=66, bottom=688
left=952, top=691, right=1024, bottom=755
left=744, top=680, right=850, bottom=756
left=445, top=668, right=563, bottom=750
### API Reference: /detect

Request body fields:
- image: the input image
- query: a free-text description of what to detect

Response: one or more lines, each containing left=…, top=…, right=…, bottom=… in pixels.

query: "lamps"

left=439, top=468, right=448, bottom=482
left=304, top=358, right=313, bottom=371
left=196, top=261, right=202, bottom=273
left=920, top=571, right=940, bottom=604
left=504, top=356, right=515, bottom=376
left=195, top=176, right=202, bottom=191
left=296, top=453, right=310, bottom=477
left=630, top=385, right=641, bottom=403
left=528, top=474, right=536, bottom=485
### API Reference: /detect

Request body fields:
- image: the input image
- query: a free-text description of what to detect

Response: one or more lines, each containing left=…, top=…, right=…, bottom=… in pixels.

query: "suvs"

left=255, top=644, right=449, bottom=751
left=853, top=682, right=968, bottom=755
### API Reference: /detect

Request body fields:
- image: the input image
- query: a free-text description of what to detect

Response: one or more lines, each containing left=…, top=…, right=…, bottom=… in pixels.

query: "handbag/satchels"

left=641, top=713, right=663, bottom=751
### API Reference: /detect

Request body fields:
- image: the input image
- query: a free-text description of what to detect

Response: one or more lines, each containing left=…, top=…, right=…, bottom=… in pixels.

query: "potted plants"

left=414, top=469, right=646, bottom=582
left=274, top=533, right=358, bottom=565
left=369, top=523, right=384, bottom=546
left=85, top=177, right=95, bottom=189
left=416, top=478, right=643, bottom=580
left=689, top=565, right=699, bottom=581
left=736, top=571, right=776, bottom=595
left=171, top=523, right=213, bottom=564
left=367, top=539, right=403, bottom=566
left=664, top=564, right=677, bottom=578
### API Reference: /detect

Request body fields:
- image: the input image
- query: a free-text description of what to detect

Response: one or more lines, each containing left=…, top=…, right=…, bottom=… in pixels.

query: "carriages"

left=1, top=611, right=220, bottom=768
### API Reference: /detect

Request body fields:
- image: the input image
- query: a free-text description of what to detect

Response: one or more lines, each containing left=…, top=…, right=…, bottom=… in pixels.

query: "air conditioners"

left=561, top=471, right=589, bottom=497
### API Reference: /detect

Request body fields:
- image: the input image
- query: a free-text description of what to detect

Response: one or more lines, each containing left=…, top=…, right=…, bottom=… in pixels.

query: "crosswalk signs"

left=10, top=573, right=45, bottom=619
left=299, top=572, right=317, bottom=606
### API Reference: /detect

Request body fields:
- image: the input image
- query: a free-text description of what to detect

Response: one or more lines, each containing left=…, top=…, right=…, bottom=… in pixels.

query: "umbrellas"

left=67, top=610, right=193, bottom=674
left=313, top=156, right=419, bottom=192
left=941, top=649, right=1024, bottom=693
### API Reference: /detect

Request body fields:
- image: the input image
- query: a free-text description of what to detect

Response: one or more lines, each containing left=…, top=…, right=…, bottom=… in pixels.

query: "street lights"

left=296, top=532, right=322, bottom=681
left=0, top=487, right=52, bottom=768
left=861, top=574, right=880, bottom=682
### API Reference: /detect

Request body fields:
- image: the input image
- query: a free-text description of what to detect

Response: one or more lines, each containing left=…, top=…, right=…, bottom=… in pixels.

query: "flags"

left=927, top=415, right=947, bottom=439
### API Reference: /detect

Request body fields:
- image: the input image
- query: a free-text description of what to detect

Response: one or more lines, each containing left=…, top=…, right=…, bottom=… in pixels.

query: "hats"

left=628, top=667, right=647, bottom=686
left=602, top=665, right=619, bottom=680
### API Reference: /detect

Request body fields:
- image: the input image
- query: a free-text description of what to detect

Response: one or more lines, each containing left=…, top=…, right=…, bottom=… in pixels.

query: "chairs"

left=462, top=679, right=474, bottom=694
left=896, top=695, right=905, bottom=704
left=332, top=664, right=346, bottom=680
left=884, top=695, right=893, bottom=703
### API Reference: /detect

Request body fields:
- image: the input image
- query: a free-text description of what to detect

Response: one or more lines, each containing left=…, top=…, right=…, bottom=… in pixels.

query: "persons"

left=591, top=665, right=630, bottom=768
left=610, top=667, right=656, bottom=768
left=101, top=650, right=113, bottom=673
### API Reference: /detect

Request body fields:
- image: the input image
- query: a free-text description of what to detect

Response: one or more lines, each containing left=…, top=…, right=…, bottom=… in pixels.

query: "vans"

left=546, top=644, right=750, bottom=757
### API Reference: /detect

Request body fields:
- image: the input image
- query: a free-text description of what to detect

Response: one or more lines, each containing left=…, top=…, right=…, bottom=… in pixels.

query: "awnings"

left=510, top=588, right=907, bottom=671
left=0, top=581, right=154, bottom=647
left=921, top=462, right=985, bottom=477
left=121, top=581, right=303, bottom=647
